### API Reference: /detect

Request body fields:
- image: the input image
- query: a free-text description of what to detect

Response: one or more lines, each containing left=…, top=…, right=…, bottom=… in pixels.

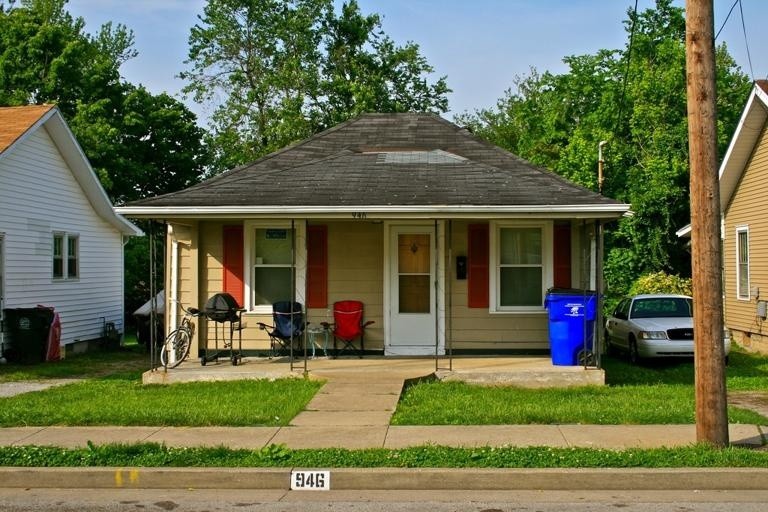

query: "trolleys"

left=200, top=293, right=247, bottom=365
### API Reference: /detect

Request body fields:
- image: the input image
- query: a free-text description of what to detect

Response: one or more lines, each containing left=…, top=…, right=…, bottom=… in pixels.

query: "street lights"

left=598, top=139, right=612, bottom=195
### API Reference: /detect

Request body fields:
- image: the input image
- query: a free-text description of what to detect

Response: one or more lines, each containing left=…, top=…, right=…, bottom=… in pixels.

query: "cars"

left=604, top=292, right=732, bottom=369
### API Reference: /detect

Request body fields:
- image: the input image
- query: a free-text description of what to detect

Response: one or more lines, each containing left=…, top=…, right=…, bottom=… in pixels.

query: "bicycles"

left=159, top=298, right=205, bottom=368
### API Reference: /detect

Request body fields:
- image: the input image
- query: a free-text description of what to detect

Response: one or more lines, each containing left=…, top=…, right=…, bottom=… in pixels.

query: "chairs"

left=256, top=301, right=376, bottom=361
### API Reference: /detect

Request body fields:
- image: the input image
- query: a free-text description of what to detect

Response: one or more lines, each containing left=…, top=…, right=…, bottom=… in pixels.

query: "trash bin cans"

left=2, top=307, right=55, bottom=365
left=544, top=288, right=597, bottom=365
